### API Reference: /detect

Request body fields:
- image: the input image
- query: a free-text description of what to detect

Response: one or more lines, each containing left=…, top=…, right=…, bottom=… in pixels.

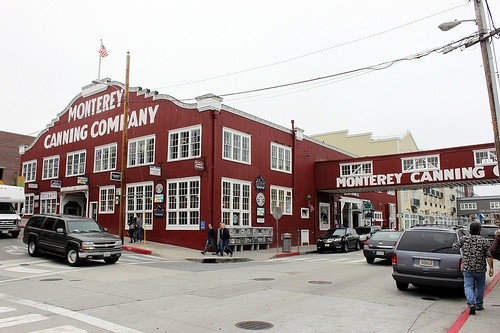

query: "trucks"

left=0, top=184, right=25, bottom=239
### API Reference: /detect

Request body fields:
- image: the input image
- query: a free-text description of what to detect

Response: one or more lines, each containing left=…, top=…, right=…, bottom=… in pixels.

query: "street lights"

left=92, top=79, right=130, bottom=245
left=438, top=18, right=500, bottom=180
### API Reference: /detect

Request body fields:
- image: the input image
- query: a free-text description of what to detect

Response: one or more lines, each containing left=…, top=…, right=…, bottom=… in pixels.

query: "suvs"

left=392, top=223, right=475, bottom=297
left=480, top=225, right=500, bottom=245
left=23, top=213, right=122, bottom=267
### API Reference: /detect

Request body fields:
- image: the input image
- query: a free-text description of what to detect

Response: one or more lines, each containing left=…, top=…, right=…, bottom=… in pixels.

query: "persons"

left=215, top=222, right=229, bottom=256
left=218, top=223, right=233, bottom=256
left=135, top=214, right=143, bottom=242
left=128, top=215, right=136, bottom=243
left=201, top=223, right=218, bottom=255
left=451, top=220, right=493, bottom=315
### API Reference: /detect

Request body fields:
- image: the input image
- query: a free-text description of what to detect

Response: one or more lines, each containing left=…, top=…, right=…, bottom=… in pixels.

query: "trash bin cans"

left=281, top=233, right=292, bottom=252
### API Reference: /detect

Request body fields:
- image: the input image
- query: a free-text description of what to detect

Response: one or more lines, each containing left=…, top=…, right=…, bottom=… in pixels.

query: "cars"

left=355, top=226, right=381, bottom=245
left=317, top=227, right=360, bottom=254
left=364, top=230, right=405, bottom=263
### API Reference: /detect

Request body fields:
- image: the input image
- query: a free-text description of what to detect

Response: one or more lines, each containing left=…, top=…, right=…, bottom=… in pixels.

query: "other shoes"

left=468, top=304, right=484, bottom=315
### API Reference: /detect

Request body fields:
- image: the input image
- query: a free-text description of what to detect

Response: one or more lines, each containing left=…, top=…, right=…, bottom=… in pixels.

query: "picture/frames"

left=301, top=207, right=309, bottom=219
left=116, top=188, right=121, bottom=195
left=154, top=194, right=164, bottom=202
left=319, top=202, right=331, bottom=231
